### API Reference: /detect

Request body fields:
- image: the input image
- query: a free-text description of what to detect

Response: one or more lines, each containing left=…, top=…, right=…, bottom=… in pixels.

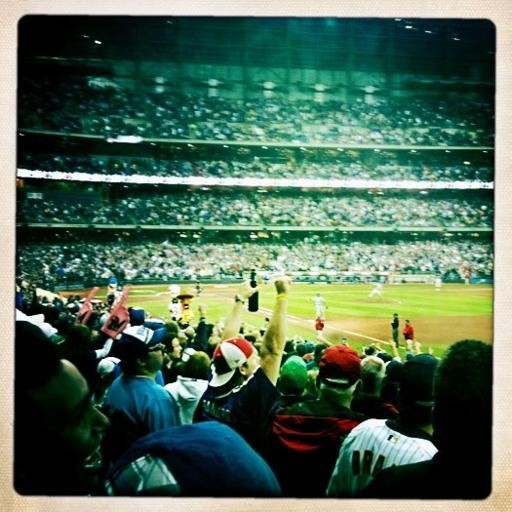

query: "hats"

left=401, top=353, right=440, bottom=410
left=111, top=326, right=165, bottom=361
left=280, top=356, right=308, bottom=391
left=98, top=419, right=282, bottom=497
left=320, top=345, right=363, bottom=389
left=209, top=339, right=254, bottom=389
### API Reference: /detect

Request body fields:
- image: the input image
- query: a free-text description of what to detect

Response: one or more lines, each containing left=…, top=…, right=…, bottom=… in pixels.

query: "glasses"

left=141, top=344, right=166, bottom=356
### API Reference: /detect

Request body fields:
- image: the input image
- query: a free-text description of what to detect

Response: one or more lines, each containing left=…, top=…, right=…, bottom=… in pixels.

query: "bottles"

left=248, top=269, right=259, bottom=312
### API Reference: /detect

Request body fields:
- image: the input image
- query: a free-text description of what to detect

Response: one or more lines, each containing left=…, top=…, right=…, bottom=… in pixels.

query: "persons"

left=14, top=271, right=493, bottom=501
left=17, top=92, right=495, bottom=288
